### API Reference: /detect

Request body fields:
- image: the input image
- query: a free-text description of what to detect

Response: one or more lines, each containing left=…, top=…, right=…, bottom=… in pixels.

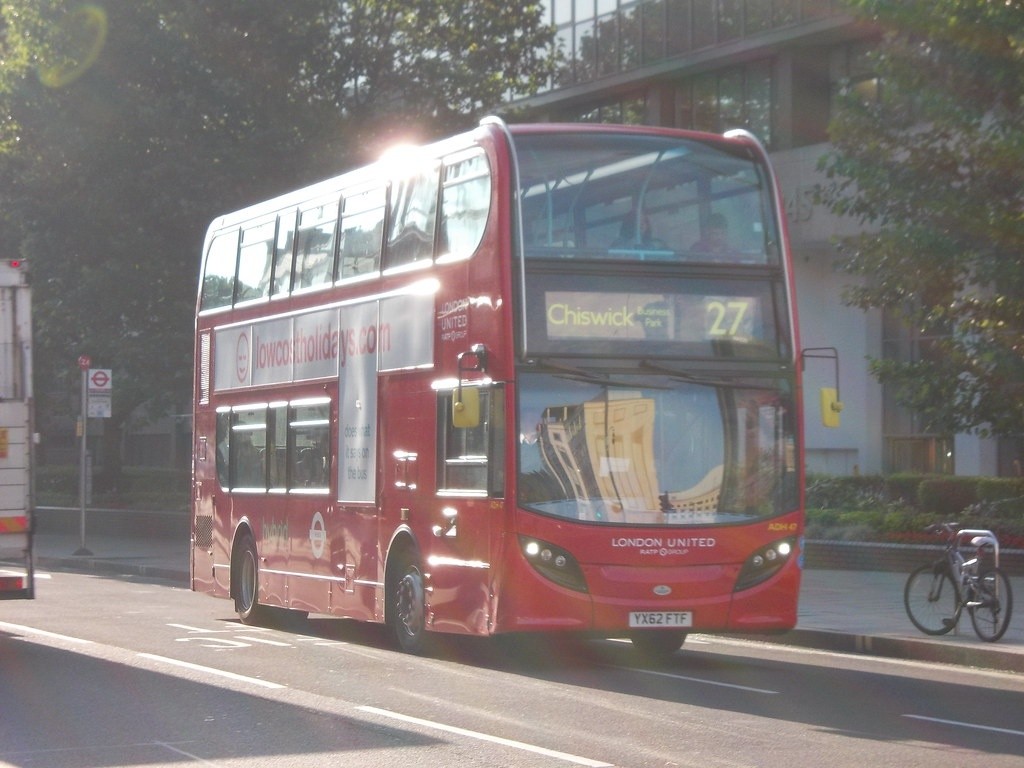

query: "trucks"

left=0, top=257, right=41, bottom=602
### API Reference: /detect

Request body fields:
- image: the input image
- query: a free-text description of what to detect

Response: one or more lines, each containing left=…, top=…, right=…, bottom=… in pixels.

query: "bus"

left=188, top=116, right=844, bottom=659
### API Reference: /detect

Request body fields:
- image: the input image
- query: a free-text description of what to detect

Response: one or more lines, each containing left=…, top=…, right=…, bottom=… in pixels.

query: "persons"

left=689, top=214, right=739, bottom=255
left=609, top=208, right=657, bottom=250
left=235, top=421, right=332, bottom=488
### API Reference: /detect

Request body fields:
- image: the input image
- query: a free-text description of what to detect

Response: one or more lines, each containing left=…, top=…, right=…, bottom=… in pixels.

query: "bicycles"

left=901, top=521, right=1012, bottom=642
